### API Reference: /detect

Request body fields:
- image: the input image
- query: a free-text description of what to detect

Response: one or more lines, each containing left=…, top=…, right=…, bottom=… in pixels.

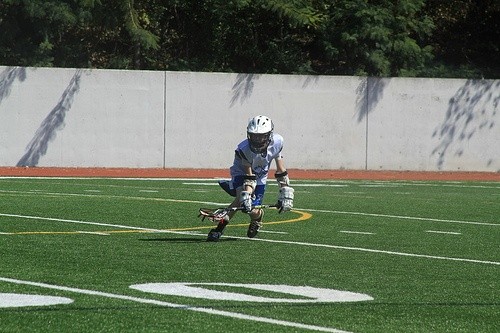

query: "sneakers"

left=207, top=228, right=224, bottom=241
left=247, top=205, right=266, bottom=238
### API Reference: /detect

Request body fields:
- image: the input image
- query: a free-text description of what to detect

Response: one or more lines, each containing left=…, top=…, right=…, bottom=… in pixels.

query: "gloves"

left=278, top=187, right=294, bottom=211
left=238, top=191, right=252, bottom=211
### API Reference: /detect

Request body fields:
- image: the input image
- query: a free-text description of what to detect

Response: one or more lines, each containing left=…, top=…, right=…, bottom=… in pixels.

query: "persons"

left=207, top=115, right=294, bottom=241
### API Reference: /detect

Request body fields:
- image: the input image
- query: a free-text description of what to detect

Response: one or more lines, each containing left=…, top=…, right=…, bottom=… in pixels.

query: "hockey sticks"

left=197, top=204, right=283, bottom=223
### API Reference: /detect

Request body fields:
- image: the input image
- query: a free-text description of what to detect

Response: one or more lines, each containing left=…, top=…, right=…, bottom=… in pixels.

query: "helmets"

left=246, top=114, right=275, bottom=134
